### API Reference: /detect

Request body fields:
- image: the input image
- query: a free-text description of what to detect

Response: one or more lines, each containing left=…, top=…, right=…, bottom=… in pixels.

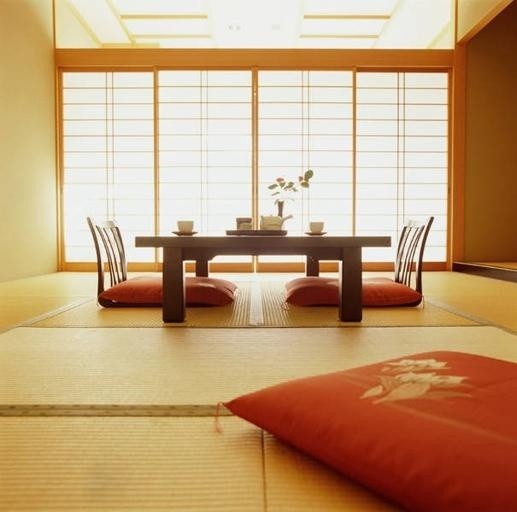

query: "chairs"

left=285, top=216, right=435, bottom=307
left=87, top=216, right=238, bottom=308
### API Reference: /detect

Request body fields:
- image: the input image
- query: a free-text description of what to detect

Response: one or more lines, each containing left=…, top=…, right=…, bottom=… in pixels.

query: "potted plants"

left=268, top=170, right=313, bottom=217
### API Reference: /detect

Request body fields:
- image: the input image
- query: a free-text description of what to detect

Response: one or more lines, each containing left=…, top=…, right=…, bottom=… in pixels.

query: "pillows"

left=285, top=276, right=424, bottom=308
left=96, top=276, right=237, bottom=306
left=213, top=350, right=517, bottom=512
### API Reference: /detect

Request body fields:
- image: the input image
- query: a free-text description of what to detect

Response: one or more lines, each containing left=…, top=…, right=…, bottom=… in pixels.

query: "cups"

left=309, top=221, right=323, bottom=233
left=177, top=220, right=193, bottom=233
left=236, top=217, right=252, bottom=230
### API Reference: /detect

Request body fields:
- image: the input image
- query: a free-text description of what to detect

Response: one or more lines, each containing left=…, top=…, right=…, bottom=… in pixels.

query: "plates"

left=305, top=232, right=327, bottom=235
left=171, top=231, right=198, bottom=236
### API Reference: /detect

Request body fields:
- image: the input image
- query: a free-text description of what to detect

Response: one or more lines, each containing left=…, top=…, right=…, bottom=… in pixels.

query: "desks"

left=135, top=235, right=391, bottom=323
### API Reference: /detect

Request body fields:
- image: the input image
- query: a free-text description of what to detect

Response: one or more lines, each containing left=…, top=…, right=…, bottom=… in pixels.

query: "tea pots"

left=260, top=215, right=292, bottom=232
